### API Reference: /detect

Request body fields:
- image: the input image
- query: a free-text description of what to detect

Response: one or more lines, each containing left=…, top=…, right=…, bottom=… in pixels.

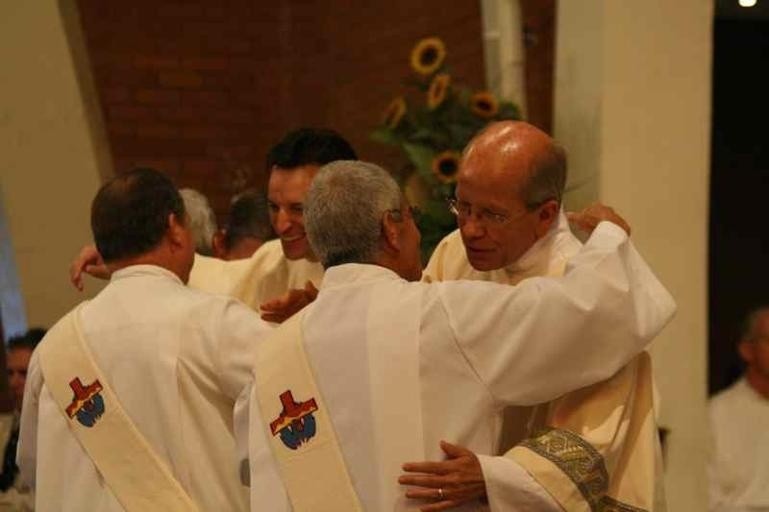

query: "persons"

left=222, top=187, right=277, bottom=261
left=0, top=328, right=47, bottom=511
left=703, top=308, right=768, bottom=512
left=230, top=160, right=678, bottom=511
left=13, top=168, right=281, bottom=511
left=70, top=126, right=360, bottom=324
left=175, top=186, right=216, bottom=257
left=258, top=118, right=667, bottom=511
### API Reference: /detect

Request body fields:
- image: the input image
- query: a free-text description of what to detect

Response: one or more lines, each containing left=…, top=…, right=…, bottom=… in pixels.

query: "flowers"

left=383, top=37, right=525, bottom=214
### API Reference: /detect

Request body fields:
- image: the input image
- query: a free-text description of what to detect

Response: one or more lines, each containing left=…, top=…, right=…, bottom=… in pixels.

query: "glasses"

left=444, top=199, right=509, bottom=226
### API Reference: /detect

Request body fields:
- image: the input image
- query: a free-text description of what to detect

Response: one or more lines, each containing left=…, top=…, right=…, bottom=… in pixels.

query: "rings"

left=437, top=488, right=444, bottom=500
